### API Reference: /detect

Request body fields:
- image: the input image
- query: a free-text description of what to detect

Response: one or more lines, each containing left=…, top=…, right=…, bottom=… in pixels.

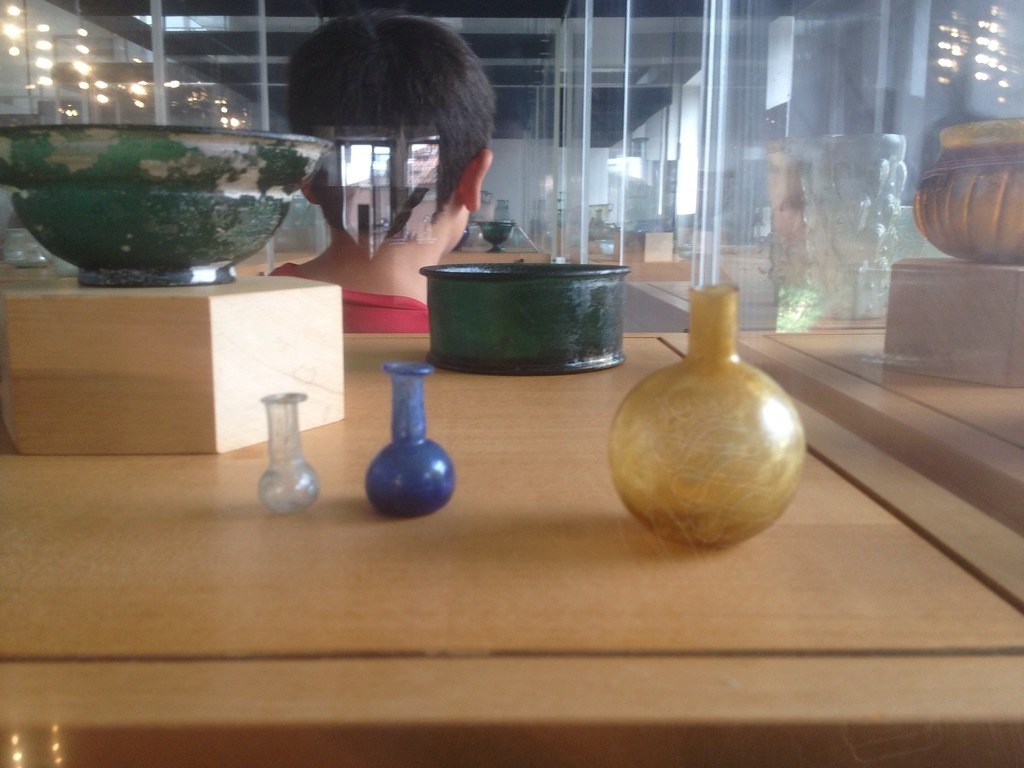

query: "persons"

left=263, top=11, right=498, bottom=333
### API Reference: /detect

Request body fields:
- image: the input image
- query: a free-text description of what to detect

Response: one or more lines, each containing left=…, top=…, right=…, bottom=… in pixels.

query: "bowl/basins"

left=0, top=112, right=344, bottom=287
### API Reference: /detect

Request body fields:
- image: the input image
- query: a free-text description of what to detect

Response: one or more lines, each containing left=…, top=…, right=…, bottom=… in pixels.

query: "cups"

left=765, top=124, right=907, bottom=320
